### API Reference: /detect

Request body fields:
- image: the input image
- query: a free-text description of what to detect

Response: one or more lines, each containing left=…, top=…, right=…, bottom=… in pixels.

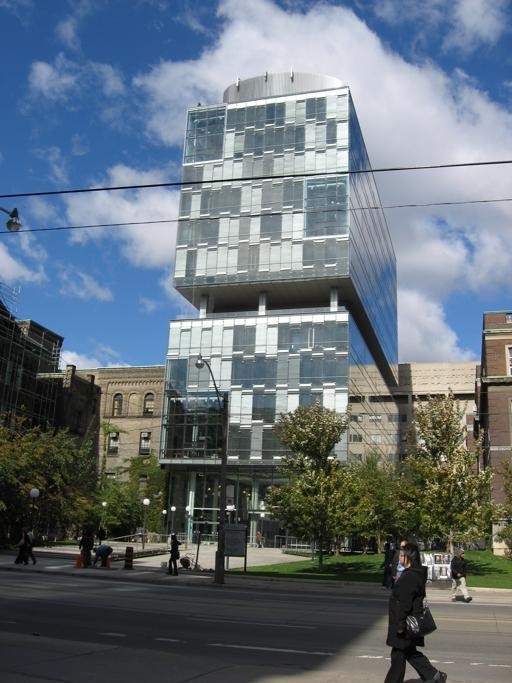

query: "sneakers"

left=465, top=596, right=472, bottom=603
left=434, top=670, right=447, bottom=683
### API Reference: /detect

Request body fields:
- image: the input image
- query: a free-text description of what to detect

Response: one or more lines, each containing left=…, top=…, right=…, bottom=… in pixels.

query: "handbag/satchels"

left=405, top=607, right=437, bottom=640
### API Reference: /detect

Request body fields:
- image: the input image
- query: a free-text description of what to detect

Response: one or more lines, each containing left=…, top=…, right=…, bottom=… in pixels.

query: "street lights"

left=30, top=488, right=41, bottom=531
left=196, top=352, right=228, bottom=552
left=259, top=512, right=265, bottom=534
left=143, top=498, right=150, bottom=528
left=162, top=505, right=191, bottom=538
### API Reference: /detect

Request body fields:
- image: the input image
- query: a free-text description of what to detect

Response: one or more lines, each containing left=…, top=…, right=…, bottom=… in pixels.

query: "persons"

left=79, top=534, right=93, bottom=565
left=380, top=543, right=395, bottom=589
left=383, top=542, right=447, bottom=683
left=449, top=548, right=472, bottom=602
left=13, top=526, right=36, bottom=569
left=165, top=534, right=181, bottom=576
left=256, top=530, right=262, bottom=548
left=93, top=545, right=113, bottom=568
left=395, top=540, right=408, bottom=582
